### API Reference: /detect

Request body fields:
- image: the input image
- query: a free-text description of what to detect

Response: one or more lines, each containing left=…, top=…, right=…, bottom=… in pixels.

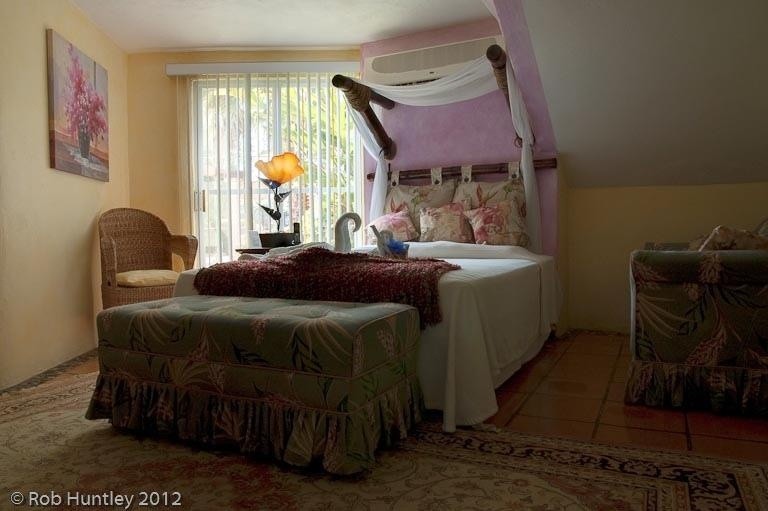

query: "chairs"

left=98, top=208, right=198, bottom=310
left=624, top=222, right=768, bottom=410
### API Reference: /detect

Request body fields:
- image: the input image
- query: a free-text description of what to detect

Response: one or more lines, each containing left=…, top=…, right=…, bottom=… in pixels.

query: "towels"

left=262, top=212, right=408, bottom=260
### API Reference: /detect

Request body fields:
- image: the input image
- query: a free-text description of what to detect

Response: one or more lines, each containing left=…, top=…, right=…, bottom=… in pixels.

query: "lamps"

left=254, top=152, right=304, bottom=247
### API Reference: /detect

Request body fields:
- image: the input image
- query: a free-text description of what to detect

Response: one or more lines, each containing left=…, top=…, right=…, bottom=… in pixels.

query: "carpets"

left=0, top=372, right=768, bottom=511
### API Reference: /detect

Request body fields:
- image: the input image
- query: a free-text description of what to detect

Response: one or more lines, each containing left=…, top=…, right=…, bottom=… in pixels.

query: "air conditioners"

left=366, top=35, right=502, bottom=85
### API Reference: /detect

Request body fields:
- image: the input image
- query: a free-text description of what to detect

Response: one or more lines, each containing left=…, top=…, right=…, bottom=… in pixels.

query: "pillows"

left=699, top=225, right=768, bottom=251
left=117, top=269, right=178, bottom=287
left=368, top=178, right=532, bottom=259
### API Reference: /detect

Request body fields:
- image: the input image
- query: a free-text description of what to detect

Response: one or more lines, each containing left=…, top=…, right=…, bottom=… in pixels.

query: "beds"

left=172, top=158, right=557, bottom=433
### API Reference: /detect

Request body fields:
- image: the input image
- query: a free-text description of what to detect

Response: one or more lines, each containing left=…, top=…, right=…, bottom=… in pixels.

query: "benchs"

left=85, top=295, right=425, bottom=475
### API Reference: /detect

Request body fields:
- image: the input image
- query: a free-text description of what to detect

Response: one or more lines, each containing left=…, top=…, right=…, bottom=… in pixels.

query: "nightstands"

left=237, top=246, right=271, bottom=254
